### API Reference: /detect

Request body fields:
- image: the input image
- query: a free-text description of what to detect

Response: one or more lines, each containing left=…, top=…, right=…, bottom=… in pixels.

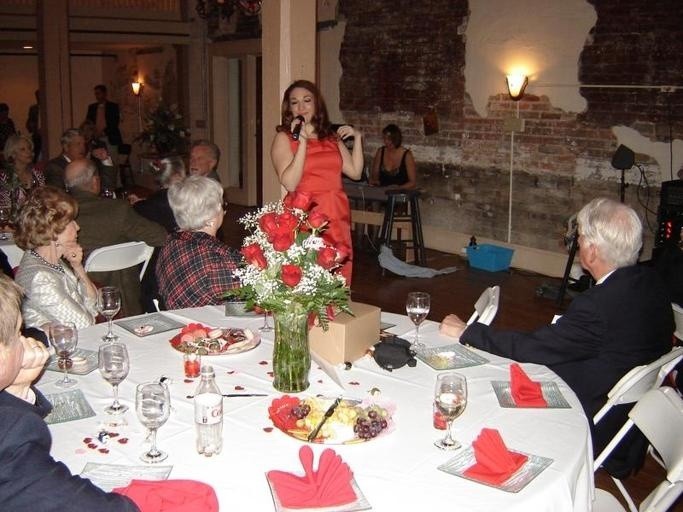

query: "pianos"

left=341, top=181, right=409, bottom=202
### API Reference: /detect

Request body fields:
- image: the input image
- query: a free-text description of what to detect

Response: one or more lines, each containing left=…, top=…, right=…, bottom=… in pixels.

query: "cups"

left=102, top=190, right=128, bottom=201
left=182, top=352, right=202, bottom=382
left=431, top=399, right=449, bottom=430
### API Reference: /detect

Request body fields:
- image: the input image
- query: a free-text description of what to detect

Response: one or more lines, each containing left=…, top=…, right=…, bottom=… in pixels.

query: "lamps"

left=131, top=81, right=142, bottom=95
left=505, top=74, right=529, bottom=101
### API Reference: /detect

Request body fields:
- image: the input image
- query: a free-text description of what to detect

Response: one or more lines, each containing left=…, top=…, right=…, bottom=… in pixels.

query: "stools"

left=554, top=221, right=597, bottom=311
left=375, top=186, right=424, bottom=278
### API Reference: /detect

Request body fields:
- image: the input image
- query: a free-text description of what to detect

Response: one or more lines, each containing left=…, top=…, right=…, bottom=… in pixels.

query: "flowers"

left=218, top=200, right=356, bottom=390
left=132, top=97, right=192, bottom=148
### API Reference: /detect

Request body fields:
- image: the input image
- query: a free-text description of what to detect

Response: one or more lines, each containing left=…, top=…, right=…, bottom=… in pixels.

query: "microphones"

left=293, top=115, right=305, bottom=140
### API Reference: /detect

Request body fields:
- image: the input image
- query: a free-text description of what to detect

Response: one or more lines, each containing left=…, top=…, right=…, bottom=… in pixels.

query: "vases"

left=152, top=131, right=175, bottom=155
left=271, top=298, right=311, bottom=393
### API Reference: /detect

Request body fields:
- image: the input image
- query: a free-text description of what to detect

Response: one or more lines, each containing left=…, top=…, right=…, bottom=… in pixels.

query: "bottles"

left=193, top=364, right=222, bottom=458
left=468, top=235, right=477, bottom=249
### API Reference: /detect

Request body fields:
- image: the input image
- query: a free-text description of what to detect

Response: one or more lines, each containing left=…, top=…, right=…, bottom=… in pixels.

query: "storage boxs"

left=466, top=243, right=515, bottom=272
left=305, top=297, right=382, bottom=367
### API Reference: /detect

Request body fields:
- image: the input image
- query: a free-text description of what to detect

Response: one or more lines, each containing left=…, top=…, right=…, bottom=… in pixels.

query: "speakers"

left=652, top=180, right=683, bottom=308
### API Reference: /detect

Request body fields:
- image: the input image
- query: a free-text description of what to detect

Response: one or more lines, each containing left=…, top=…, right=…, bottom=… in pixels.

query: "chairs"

left=591, top=347, right=683, bottom=511
left=455, top=286, right=499, bottom=340
left=669, top=302, right=683, bottom=344
left=84, top=240, right=155, bottom=284
left=590, top=386, right=682, bottom=511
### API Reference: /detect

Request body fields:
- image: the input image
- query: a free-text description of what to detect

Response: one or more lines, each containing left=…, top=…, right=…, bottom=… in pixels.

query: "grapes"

left=354, top=411, right=387, bottom=438
left=292, top=405, right=310, bottom=419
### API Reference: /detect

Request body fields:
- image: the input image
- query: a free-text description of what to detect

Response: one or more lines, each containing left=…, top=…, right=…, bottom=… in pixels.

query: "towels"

left=462, top=428, right=527, bottom=488
left=109, top=479, right=219, bottom=512
left=270, top=445, right=357, bottom=509
left=508, top=362, right=548, bottom=407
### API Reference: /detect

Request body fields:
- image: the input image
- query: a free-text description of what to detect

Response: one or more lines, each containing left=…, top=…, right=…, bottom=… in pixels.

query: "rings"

left=71, top=253, right=76, bottom=257
left=31, top=345, right=42, bottom=351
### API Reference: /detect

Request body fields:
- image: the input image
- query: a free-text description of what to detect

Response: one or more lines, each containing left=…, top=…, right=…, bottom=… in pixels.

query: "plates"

left=41, top=389, right=96, bottom=425
left=43, top=347, right=102, bottom=374
left=171, top=326, right=261, bottom=358
left=380, top=322, right=396, bottom=330
left=113, top=311, right=186, bottom=338
left=224, top=301, right=258, bottom=317
left=435, top=441, right=556, bottom=494
left=78, top=461, right=173, bottom=493
left=413, top=341, right=489, bottom=370
left=264, top=462, right=374, bottom=511
left=489, top=380, right=572, bottom=408
left=270, top=395, right=393, bottom=444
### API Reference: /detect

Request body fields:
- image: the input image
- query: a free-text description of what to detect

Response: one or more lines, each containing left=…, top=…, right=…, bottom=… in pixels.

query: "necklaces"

left=31, top=250, right=64, bottom=273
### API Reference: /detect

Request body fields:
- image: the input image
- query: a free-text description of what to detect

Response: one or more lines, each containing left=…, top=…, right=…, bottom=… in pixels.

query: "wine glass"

left=258, top=307, right=275, bottom=332
left=406, top=292, right=431, bottom=353
left=95, top=286, right=122, bottom=343
left=0, top=208, right=9, bottom=240
left=432, top=371, right=468, bottom=452
left=96, top=343, right=130, bottom=415
left=47, top=320, right=79, bottom=388
left=134, top=380, right=171, bottom=463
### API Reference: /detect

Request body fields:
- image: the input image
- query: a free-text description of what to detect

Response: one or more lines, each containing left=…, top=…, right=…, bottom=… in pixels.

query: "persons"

left=438, top=198, right=674, bottom=480
left=360, top=125, right=417, bottom=257
left=126, top=157, right=223, bottom=313
left=187, top=141, right=218, bottom=180
left=0, top=273, right=139, bottom=511
left=155, top=173, right=244, bottom=310
left=0, top=137, right=45, bottom=232
left=14, top=185, right=101, bottom=332
left=0, top=84, right=124, bottom=197
left=270, top=81, right=364, bottom=297
left=63, top=158, right=169, bottom=324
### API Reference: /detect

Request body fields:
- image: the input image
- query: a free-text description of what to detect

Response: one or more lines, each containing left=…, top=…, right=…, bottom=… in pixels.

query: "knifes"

left=304, top=394, right=345, bottom=442
left=184, top=393, right=269, bottom=399
